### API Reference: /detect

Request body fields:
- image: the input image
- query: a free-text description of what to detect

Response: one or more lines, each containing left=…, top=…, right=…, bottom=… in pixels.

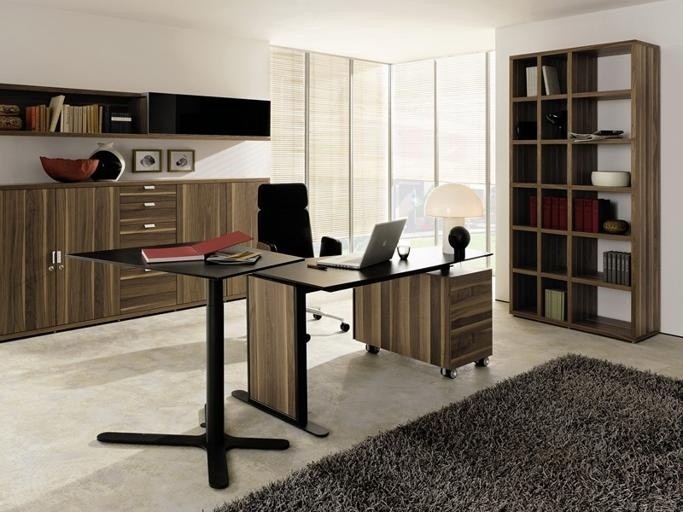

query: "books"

left=0, top=94, right=141, bottom=134
left=140, top=229, right=262, bottom=265
left=526, top=65, right=558, bottom=99
left=544, top=286, right=568, bottom=324
left=602, top=250, right=633, bottom=289
left=528, top=193, right=611, bottom=234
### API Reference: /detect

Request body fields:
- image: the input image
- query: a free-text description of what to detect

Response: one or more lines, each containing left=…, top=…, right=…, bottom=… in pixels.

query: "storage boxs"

left=544, top=288, right=567, bottom=322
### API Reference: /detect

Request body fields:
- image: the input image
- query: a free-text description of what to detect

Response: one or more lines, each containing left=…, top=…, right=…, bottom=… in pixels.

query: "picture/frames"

left=133, top=149, right=162, bottom=172
left=168, top=149, right=195, bottom=172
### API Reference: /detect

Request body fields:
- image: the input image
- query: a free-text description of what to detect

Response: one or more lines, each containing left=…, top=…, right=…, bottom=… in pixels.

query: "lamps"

left=423, top=183, right=484, bottom=254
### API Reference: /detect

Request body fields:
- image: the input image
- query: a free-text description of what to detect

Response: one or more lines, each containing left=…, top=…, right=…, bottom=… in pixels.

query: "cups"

left=396, top=246, right=411, bottom=260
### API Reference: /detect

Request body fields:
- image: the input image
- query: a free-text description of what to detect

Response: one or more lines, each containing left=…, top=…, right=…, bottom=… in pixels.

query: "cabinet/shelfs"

left=508, top=38, right=660, bottom=343
left=0, top=82, right=271, bottom=141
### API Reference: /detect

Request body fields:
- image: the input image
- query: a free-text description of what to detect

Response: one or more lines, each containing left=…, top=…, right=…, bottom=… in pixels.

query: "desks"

left=228, top=250, right=494, bottom=437
left=65, top=240, right=290, bottom=489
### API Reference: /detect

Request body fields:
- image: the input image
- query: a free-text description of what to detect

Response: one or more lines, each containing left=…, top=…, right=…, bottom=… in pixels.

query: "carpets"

left=208, top=352, right=683, bottom=511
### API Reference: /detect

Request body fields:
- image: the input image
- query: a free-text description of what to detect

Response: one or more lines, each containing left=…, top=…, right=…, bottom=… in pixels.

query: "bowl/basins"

left=39, top=155, right=99, bottom=183
left=591, top=171, right=630, bottom=188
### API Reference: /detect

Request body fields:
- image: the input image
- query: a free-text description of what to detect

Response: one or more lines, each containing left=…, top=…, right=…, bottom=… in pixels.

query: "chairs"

left=256, top=182, right=349, bottom=342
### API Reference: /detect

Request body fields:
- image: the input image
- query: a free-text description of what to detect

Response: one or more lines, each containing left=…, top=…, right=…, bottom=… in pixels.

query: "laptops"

left=316, top=217, right=407, bottom=270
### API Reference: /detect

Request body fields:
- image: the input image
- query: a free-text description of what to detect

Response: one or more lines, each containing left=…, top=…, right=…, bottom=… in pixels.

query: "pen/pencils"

left=308, top=264, right=328, bottom=272
